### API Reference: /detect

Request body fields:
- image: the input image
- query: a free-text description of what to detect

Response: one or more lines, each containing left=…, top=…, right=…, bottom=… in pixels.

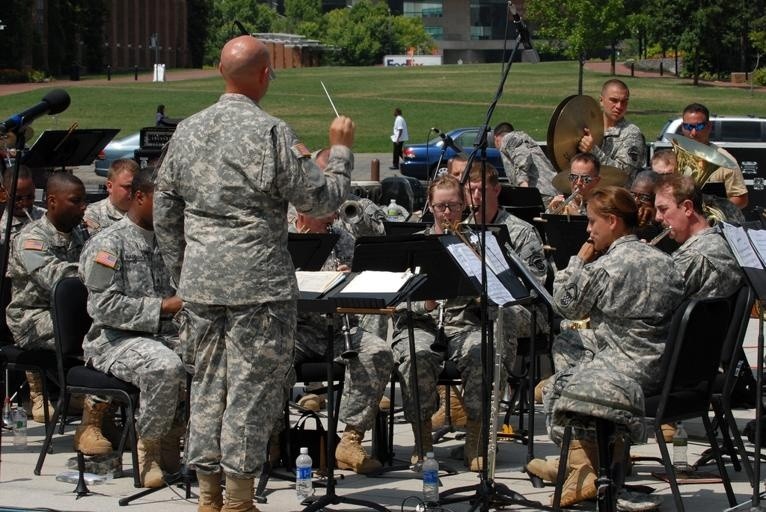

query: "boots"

left=57, top=389, right=83, bottom=415
left=223, top=477, right=262, bottom=512
left=134, top=433, right=161, bottom=488
left=526, top=453, right=568, bottom=482
left=655, top=421, right=676, bottom=443
left=23, top=370, right=55, bottom=421
left=547, top=441, right=605, bottom=505
left=294, top=379, right=327, bottom=412
left=265, top=429, right=283, bottom=469
left=75, top=392, right=114, bottom=456
left=431, top=383, right=470, bottom=429
left=610, top=437, right=632, bottom=474
left=465, top=419, right=487, bottom=471
left=411, top=419, right=438, bottom=465
left=162, top=436, right=182, bottom=472
left=195, top=470, right=220, bottom=512
left=338, top=426, right=385, bottom=473
left=378, top=390, right=393, bottom=411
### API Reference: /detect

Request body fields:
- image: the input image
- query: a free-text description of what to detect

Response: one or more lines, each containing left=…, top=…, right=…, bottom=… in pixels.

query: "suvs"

left=656, top=114, right=766, bottom=160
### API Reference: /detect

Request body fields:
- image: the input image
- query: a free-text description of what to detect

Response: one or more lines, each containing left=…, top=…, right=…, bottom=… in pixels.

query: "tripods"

left=424, top=174, right=546, bottom=512
left=118, top=370, right=204, bottom=507
left=300, top=334, right=388, bottom=512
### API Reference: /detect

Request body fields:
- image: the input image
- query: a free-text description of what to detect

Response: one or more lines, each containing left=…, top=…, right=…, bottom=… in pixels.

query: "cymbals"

left=551, top=166, right=627, bottom=192
left=547, top=94, right=604, bottom=174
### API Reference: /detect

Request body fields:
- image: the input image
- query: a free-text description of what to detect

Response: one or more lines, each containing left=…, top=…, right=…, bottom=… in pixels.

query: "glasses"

left=567, top=171, right=600, bottom=186
left=680, top=120, right=710, bottom=132
left=630, top=189, right=657, bottom=203
left=3, top=184, right=35, bottom=203
left=430, top=201, right=464, bottom=214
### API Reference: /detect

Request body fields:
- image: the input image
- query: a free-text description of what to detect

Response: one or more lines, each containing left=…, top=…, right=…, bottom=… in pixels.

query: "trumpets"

left=544, top=185, right=583, bottom=215
left=340, top=200, right=365, bottom=237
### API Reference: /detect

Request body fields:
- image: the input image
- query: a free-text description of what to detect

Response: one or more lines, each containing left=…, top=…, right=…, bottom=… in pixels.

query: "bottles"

left=672, top=424, right=688, bottom=472
left=294, top=446, right=314, bottom=502
left=385, top=198, right=400, bottom=223
left=421, top=451, right=440, bottom=504
left=8, top=404, right=29, bottom=451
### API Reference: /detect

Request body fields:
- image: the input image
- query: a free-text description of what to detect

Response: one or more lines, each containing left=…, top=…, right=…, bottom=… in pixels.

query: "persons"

left=2, top=36, right=766, bottom=512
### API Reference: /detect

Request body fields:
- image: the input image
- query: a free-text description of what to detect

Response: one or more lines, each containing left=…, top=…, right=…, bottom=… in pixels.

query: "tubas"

left=663, top=134, right=744, bottom=227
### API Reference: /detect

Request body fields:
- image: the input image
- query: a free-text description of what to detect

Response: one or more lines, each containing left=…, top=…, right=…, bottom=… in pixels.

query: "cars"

left=399, top=126, right=550, bottom=186
left=95, top=128, right=145, bottom=183
left=3, top=141, right=31, bottom=167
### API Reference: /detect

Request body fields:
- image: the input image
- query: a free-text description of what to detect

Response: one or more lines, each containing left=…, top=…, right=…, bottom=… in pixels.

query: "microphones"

left=429, top=123, right=464, bottom=154
left=0, top=86, right=73, bottom=137
left=514, top=12, right=541, bottom=67
